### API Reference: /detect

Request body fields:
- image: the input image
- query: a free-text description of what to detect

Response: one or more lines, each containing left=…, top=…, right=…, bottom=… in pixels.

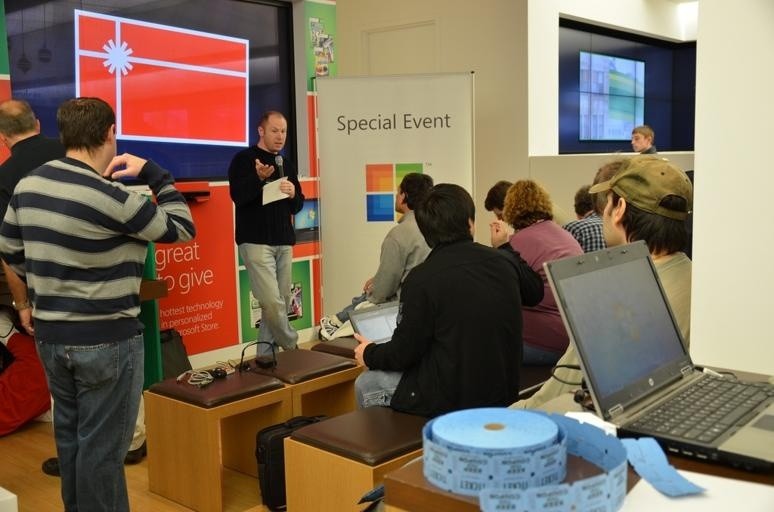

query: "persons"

left=628, top=123, right=656, bottom=155
left=0, top=98, right=197, bottom=511
left=228, top=112, right=304, bottom=357
left=0, top=100, right=147, bottom=475
left=321, top=158, right=691, bottom=408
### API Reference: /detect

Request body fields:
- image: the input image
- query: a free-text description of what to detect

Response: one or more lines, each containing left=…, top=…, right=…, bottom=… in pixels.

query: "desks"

left=383, top=366, right=774, bottom=510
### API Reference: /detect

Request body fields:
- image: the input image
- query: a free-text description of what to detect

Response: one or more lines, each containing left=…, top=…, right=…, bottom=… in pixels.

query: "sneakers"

left=321, top=314, right=339, bottom=340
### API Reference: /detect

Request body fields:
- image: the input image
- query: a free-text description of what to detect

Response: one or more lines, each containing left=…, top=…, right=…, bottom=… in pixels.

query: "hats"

left=589, top=153, right=694, bottom=222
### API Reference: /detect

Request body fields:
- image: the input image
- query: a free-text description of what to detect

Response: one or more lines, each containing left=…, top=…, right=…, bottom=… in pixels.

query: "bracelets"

left=11, top=299, right=31, bottom=313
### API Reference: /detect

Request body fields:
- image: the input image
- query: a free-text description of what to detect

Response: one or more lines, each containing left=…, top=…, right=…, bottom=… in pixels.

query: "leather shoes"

left=124, top=438, right=147, bottom=465
left=42, top=457, right=60, bottom=478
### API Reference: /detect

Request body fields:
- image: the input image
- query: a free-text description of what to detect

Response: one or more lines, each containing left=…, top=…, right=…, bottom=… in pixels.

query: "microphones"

left=271, top=156, right=290, bottom=178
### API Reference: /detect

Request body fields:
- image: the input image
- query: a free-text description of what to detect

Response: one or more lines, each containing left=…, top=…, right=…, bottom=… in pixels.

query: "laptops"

left=350, top=299, right=402, bottom=346
left=541, top=239, right=770, bottom=482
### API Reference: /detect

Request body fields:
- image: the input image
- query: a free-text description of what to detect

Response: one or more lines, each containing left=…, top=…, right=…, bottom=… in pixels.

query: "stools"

left=143, top=365, right=293, bottom=512
left=284, top=405, right=432, bottom=512
left=311, top=335, right=362, bottom=359
left=234, top=349, right=365, bottom=428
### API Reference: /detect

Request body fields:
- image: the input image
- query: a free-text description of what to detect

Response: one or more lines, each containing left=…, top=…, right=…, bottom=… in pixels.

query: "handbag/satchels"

left=256, top=415, right=334, bottom=512
left=161, top=327, right=193, bottom=381
